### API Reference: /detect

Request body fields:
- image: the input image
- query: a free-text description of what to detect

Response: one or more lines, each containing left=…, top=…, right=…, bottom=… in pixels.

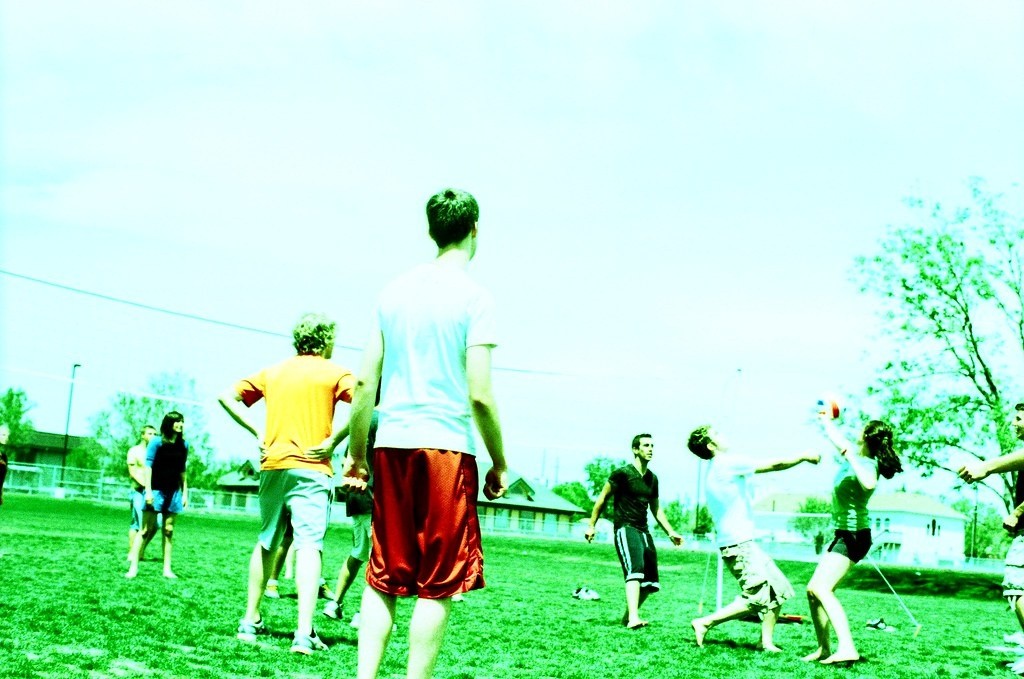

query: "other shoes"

left=324, top=600, right=343, bottom=618
left=572, top=586, right=598, bottom=602
left=265, top=580, right=279, bottom=598
left=320, top=583, right=334, bottom=601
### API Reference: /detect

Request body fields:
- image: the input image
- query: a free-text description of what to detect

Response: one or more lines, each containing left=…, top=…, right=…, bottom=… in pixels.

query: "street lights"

left=59, top=363, right=82, bottom=486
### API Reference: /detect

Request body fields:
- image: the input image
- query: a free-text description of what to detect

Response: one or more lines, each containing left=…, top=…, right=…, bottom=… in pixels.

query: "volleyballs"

left=811, top=397, right=839, bottom=424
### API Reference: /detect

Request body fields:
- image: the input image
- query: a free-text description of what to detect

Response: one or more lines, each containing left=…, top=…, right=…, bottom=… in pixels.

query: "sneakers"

left=289, top=630, right=327, bottom=656
left=237, top=615, right=266, bottom=641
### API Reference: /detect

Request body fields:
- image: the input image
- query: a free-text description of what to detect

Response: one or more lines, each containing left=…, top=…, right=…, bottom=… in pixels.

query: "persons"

left=583, top=434, right=684, bottom=628
left=217, top=189, right=508, bottom=679
left=126, top=411, right=189, bottom=579
left=799, top=401, right=903, bottom=663
left=958, top=403, right=1024, bottom=677
left=681, top=424, right=820, bottom=654
